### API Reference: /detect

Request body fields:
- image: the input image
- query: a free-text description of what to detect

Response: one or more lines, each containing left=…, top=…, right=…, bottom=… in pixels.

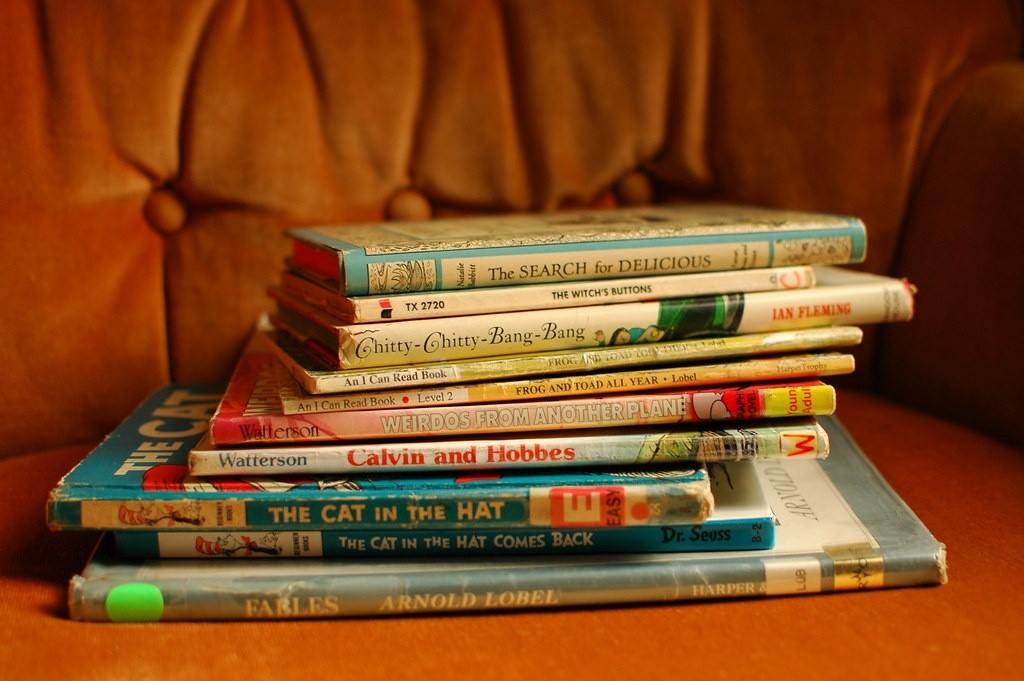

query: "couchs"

left=0, top=0, right=1024, bottom=680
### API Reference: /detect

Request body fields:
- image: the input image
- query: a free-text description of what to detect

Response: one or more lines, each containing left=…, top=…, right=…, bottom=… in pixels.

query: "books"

left=44, top=203, right=948, bottom=621
